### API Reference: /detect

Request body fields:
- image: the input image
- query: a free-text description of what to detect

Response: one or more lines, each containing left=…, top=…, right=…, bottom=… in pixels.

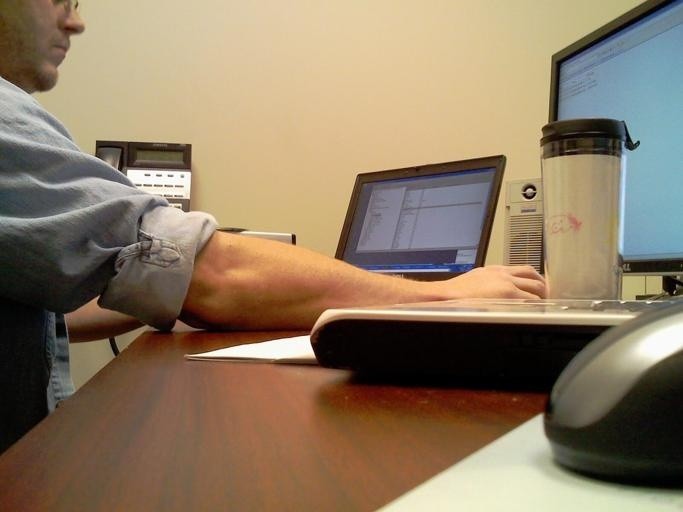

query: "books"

left=184, top=334, right=318, bottom=364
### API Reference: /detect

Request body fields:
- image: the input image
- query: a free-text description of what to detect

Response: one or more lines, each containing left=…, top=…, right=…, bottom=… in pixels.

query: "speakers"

left=503, top=178, right=544, bottom=275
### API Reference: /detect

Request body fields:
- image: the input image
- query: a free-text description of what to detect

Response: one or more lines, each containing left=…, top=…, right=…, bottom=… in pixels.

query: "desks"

left=1, top=315, right=551, bottom=511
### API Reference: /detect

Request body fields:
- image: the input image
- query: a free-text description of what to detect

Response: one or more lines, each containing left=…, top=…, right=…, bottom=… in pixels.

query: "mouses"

left=543, top=300, right=683, bottom=491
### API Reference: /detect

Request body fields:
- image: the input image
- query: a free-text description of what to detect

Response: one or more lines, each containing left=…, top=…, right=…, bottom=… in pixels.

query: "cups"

left=539, top=117, right=640, bottom=302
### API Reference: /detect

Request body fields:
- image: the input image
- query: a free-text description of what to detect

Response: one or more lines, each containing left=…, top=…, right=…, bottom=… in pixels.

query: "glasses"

left=52, top=0, right=81, bottom=16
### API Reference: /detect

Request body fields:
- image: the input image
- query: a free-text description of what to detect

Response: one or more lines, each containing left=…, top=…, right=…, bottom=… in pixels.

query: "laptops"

left=334, top=154, right=507, bottom=282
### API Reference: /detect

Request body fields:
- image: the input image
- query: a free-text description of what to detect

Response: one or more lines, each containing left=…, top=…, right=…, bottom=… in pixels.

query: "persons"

left=0, top=1, right=547, bottom=466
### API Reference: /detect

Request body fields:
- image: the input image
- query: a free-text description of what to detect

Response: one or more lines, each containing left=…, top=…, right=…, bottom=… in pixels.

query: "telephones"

left=94, top=139, right=192, bottom=214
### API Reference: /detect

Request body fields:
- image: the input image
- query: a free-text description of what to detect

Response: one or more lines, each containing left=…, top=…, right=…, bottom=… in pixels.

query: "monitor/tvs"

left=549, top=0, right=683, bottom=299
